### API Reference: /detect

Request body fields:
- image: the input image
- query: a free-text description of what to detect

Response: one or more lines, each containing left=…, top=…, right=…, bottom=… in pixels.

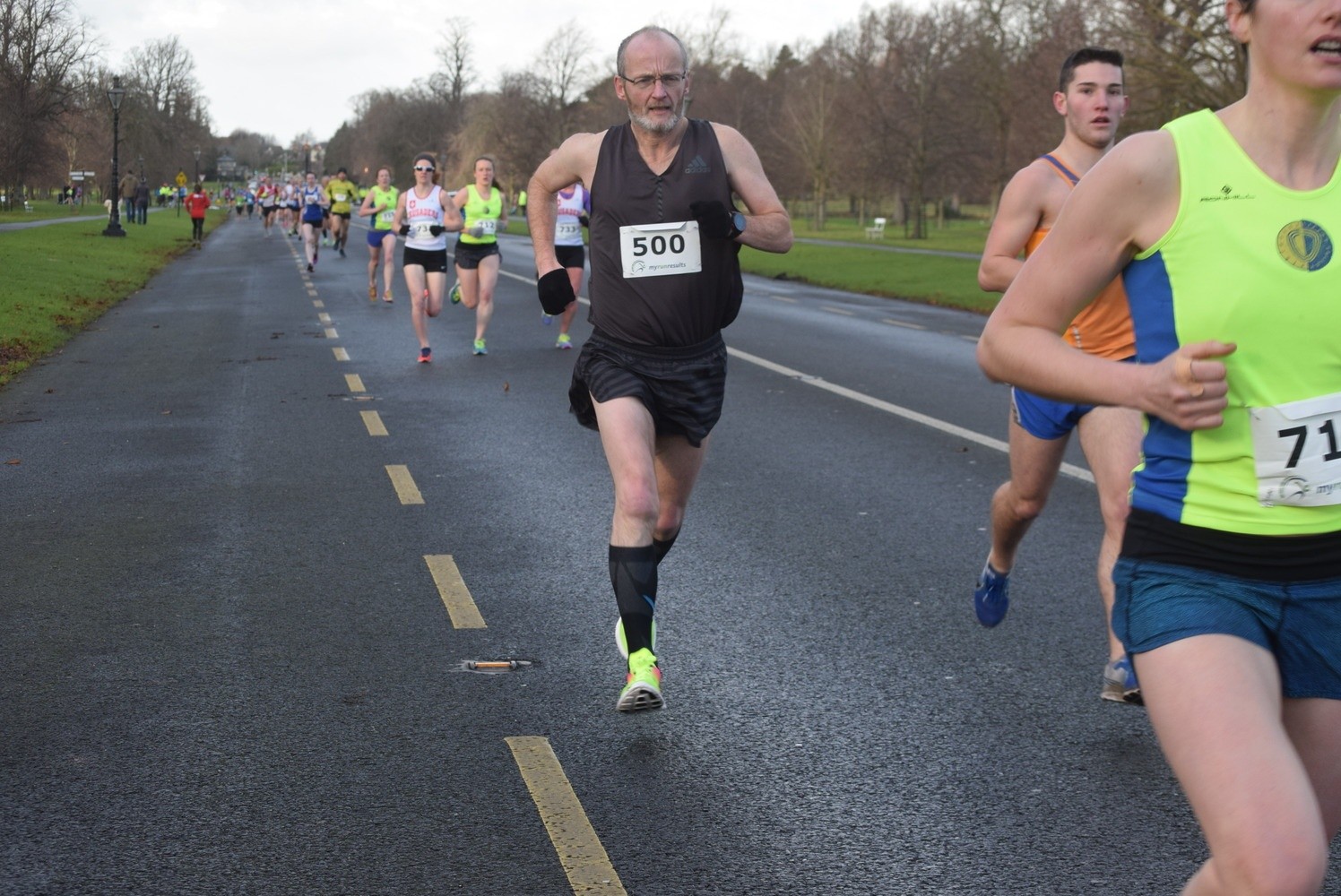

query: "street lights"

left=102, top=74, right=128, bottom=237
left=138, top=155, right=144, bottom=177
left=193, top=143, right=201, bottom=184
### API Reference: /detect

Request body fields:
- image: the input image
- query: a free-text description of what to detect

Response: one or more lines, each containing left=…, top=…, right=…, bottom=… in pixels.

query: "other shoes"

left=191, top=240, right=202, bottom=250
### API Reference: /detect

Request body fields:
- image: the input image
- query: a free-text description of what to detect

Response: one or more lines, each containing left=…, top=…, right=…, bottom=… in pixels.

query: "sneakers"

left=262, top=223, right=347, bottom=272
left=473, top=339, right=487, bottom=355
left=541, top=309, right=553, bottom=325
left=974, top=546, right=1011, bottom=629
left=557, top=333, right=573, bottom=350
left=369, top=279, right=378, bottom=302
left=382, top=289, right=394, bottom=303
left=448, top=278, right=463, bottom=305
left=422, top=288, right=429, bottom=316
left=616, top=647, right=667, bottom=714
left=418, top=347, right=432, bottom=363
left=1100, top=655, right=1146, bottom=706
left=615, top=614, right=657, bottom=659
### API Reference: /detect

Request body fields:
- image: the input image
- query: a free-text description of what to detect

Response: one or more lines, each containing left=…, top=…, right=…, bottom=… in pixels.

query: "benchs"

left=866, top=217, right=886, bottom=241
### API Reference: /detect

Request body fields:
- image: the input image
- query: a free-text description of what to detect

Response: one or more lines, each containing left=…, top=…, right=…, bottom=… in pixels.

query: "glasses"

left=619, top=71, right=686, bottom=89
left=414, top=166, right=435, bottom=172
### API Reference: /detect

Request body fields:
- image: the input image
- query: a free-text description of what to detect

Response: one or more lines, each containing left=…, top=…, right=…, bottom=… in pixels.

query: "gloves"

left=430, top=225, right=446, bottom=237
left=347, top=190, right=353, bottom=198
left=330, top=199, right=335, bottom=204
left=400, top=224, right=410, bottom=236
left=691, top=199, right=732, bottom=240
left=578, top=216, right=588, bottom=227
left=470, top=227, right=484, bottom=239
left=537, top=268, right=576, bottom=314
left=498, top=222, right=506, bottom=232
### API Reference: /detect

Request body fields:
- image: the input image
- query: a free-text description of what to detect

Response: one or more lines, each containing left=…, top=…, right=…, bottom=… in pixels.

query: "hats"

left=338, top=167, right=347, bottom=174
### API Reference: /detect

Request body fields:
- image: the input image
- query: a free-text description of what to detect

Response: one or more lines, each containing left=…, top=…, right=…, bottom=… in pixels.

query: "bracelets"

left=728, top=211, right=745, bottom=241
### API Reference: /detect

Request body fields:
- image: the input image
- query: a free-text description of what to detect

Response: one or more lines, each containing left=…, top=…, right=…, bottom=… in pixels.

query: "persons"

left=977, top=0, right=1341, bottom=896
left=536, top=149, right=591, bottom=349
left=185, top=184, right=210, bottom=250
left=118, top=170, right=152, bottom=225
left=511, top=187, right=526, bottom=216
left=358, top=168, right=400, bottom=301
left=155, top=183, right=213, bottom=208
left=24, top=194, right=28, bottom=201
left=64, top=183, right=82, bottom=205
left=224, top=183, right=255, bottom=220
left=975, top=47, right=1144, bottom=703
left=444, top=156, right=508, bottom=358
left=391, top=153, right=464, bottom=363
left=255, top=168, right=370, bottom=257
left=526, top=25, right=794, bottom=713
left=298, top=171, right=330, bottom=272
